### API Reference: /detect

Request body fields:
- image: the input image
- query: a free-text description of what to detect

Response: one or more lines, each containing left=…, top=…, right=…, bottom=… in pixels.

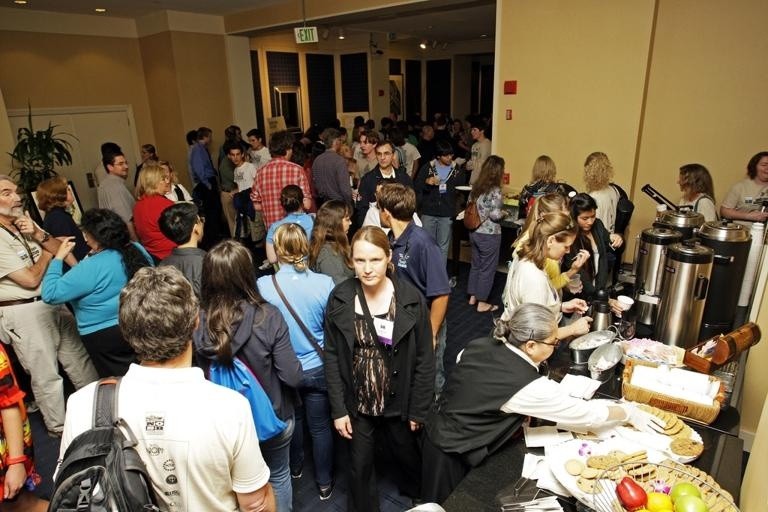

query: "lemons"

left=645, top=492, right=672, bottom=512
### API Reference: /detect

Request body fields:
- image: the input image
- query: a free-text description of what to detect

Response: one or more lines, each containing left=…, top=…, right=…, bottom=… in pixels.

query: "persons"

left=217, top=126, right=248, bottom=156
left=354, top=131, right=381, bottom=193
left=358, top=140, right=413, bottom=217
left=678, top=164, right=720, bottom=224
left=220, top=138, right=248, bottom=238
left=309, top=202, right=353, bottom=282
left=584, top=152, right=623, bottom=235
left=419, top=110, right=481, bottom=161
left=466, top=156, right=512, bottom=313
left=322, top=227, right=435, bottom=512
left=377, top=183, right=449, bottom=404
left=0, top=174, right=98, bottom=437
left=256, top=222, right=338, bottom=499
left=43, top=209, right=155, bottom=378
left=192, top=128, right=222, bottom=241
left=229, top=148, right=259, bottom=240
left=518, top=156, right=555, bottom=220
left=311, top=131, right=353, bottom=209
left=290, top=127, right=322, bottom=182
left=720, top=150, right=767, bottom=221
left=133, top=167, right=180, bottom=262
left=135, top=146, right=157, bottom=180
left=98, top=152, right=139, bottom=241
left=266, top=185, right=313, bottom=264
left=59, top=266, right=277, bottom=512
left=416, top=144, right=460, bottom=266
left=330, top=116, right=375, bottom=158
left=186, top=130, right=197, bottom=182
left=1, top=343, right=51, bottom=511
left=100, top=143, right=121, bottom=155
left=244, top=128, right=273, bottom=168
left=513, top=192, right=591, bottom=289
left=158, top=160, right=196, bottom=206
left=423, top=303, right=665, bottom=504
left=502, top=212, right=592, bottom=340
left=380, top=117, right=421, bottom=178
left=253, top=133, right=313, bottom=228
left=569, top=193, right=622, bottom=300
left=470, top=124, right=491, bottom=183
left=193, top=239, right=304, bottom=511
left=156, top=201, right=206, bottom=293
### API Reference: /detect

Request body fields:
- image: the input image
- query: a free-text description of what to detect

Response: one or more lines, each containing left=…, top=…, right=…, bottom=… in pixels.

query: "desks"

left=444, top=351, right=744, bottom=512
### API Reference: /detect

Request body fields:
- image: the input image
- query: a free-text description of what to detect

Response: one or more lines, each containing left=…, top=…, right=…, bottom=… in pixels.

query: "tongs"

left=500, top=496, right=563, bottom=512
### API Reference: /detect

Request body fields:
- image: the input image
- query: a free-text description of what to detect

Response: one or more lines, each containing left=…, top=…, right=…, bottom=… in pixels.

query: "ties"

left=206, top=148, right=219, bottom=175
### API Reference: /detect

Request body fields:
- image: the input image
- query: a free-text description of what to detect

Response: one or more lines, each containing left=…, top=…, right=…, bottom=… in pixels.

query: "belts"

left=1, top=295, right=42, bottom=306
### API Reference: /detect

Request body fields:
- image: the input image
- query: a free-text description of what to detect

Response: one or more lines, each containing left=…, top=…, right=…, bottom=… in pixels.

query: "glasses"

left=109, top=162, right=129, bottom=167
left=534, top=337, right=561, bottom=348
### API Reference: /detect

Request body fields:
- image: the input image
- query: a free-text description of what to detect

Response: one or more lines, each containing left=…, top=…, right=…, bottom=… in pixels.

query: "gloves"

left=621, top=402, right=666, bottom=433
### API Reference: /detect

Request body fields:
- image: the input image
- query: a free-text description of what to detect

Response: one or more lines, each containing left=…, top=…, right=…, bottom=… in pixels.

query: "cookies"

left=625, top=403, right=691, bottom=438
left=670, top=440, right=702, bottom=456
left=581, top=456, right=622, bottom=479
left=577, top=479, right=604, bottom=495
left=566, top=459, right=583, bottom=475
left=619, top=449, right=674, bottom=485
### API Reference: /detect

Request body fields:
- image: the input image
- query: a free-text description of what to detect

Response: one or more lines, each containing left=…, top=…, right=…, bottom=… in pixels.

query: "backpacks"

left=209, top=358, right=286, bottom=442
left=467, top=191, right=489, bottom=232
left=47, top=376, right=159, bottom=512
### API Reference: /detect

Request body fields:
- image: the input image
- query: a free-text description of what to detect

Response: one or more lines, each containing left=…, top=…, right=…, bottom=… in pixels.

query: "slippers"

left=476, top=305, right=502, bottom=314
left=469, top=303, right=479, bottom=307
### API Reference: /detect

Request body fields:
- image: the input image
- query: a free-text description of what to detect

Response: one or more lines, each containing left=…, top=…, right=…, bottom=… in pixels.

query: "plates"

left=576, top=399, right=703, bottom=463
left=544, top=432, right=685, bottom=511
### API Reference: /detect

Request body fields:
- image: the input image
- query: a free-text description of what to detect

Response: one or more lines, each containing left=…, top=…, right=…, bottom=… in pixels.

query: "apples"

left=617, top=477, right=647, bottom=510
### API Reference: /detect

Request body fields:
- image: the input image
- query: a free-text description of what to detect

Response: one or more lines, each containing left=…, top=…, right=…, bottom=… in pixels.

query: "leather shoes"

left=320, top=478, right=338, bottom=500
left=292, top=464, right=304, bottom=479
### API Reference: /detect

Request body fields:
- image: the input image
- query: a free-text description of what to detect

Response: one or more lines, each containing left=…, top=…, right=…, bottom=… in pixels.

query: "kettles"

left=589, top=300, right=613, bottom=331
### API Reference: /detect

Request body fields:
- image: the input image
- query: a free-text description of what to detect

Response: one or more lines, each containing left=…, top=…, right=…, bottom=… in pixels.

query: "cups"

left=732, top=220, right=765, bottom=306
left=616, top=295, right=634, bottom=318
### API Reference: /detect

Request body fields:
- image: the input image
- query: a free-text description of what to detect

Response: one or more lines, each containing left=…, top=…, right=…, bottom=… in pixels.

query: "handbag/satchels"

left=608, top=179, right=636, bottom=234
